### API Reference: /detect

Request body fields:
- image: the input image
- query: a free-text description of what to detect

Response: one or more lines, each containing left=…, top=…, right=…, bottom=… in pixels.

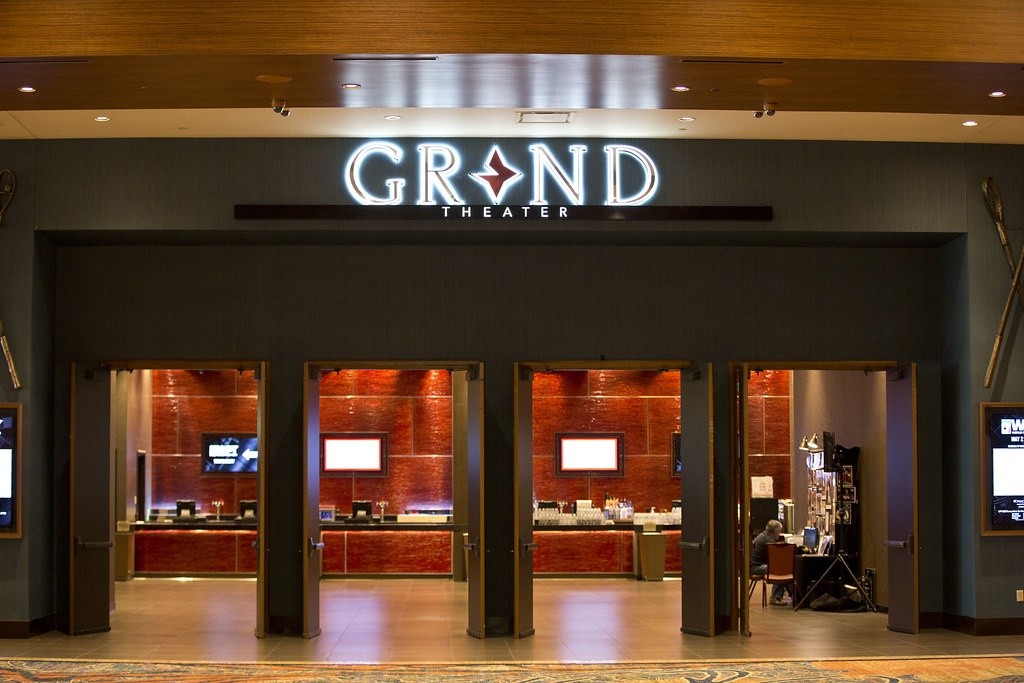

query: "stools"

left=748, top=574, right=769, bottom=608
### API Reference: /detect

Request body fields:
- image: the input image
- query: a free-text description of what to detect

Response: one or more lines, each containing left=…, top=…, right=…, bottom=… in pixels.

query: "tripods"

left=791, top=462, right=879, bottom=612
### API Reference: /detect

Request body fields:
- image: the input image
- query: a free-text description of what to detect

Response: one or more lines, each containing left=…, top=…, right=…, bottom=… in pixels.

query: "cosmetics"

left=536, top=506, right=602, bottom=525
left=604, top=492, right=634, bottom=519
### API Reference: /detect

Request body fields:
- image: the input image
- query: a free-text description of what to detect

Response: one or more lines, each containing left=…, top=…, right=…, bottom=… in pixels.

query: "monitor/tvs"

left=803, top=529, right=820, bottom=549
left=671, top=432, right=683, bottom=478
left=555, top=433, right=624, bottom=478
left=823, top=430, right=836, bottom=472
left=319, top=432, right=389, bottom=478
left=201, top=432, right=258, bottom=478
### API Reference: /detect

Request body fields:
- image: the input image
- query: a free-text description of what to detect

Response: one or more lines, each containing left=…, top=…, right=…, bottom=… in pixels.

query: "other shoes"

left=769, top=596, right=787, bottom=606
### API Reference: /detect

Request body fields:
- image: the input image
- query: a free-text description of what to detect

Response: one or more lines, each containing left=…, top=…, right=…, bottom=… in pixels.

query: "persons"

left=750, top=519, right=787, bottom=606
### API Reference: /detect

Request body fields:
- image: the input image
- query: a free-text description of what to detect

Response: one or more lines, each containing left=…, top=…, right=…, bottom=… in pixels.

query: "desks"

left=793, top=554, right=847, bottom=609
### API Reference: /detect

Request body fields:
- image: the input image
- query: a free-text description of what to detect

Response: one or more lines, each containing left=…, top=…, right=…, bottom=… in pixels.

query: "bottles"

left=533, top=492, right=682, bottom=526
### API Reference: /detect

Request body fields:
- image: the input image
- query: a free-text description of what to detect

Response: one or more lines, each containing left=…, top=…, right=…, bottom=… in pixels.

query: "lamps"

left=798, top=437, right=809, bottom=451
left=807, top=434, right=818, bottom=448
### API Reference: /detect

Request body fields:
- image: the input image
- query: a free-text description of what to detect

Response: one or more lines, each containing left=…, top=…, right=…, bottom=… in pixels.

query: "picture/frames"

left=979, top=403, right=1023, bottom=536
left=0, top=402, right=22, bottom=539
left=198, top=432, right=258, bottom=479
left=670, top=433, right=683, bottom=478
left=553, top=432, right=626, bottom=477
left=317, top=431, right=389, bottom=477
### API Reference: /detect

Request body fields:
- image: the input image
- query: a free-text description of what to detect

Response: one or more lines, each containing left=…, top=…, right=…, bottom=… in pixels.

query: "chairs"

left=764, top=543, right=799, bottom=609
